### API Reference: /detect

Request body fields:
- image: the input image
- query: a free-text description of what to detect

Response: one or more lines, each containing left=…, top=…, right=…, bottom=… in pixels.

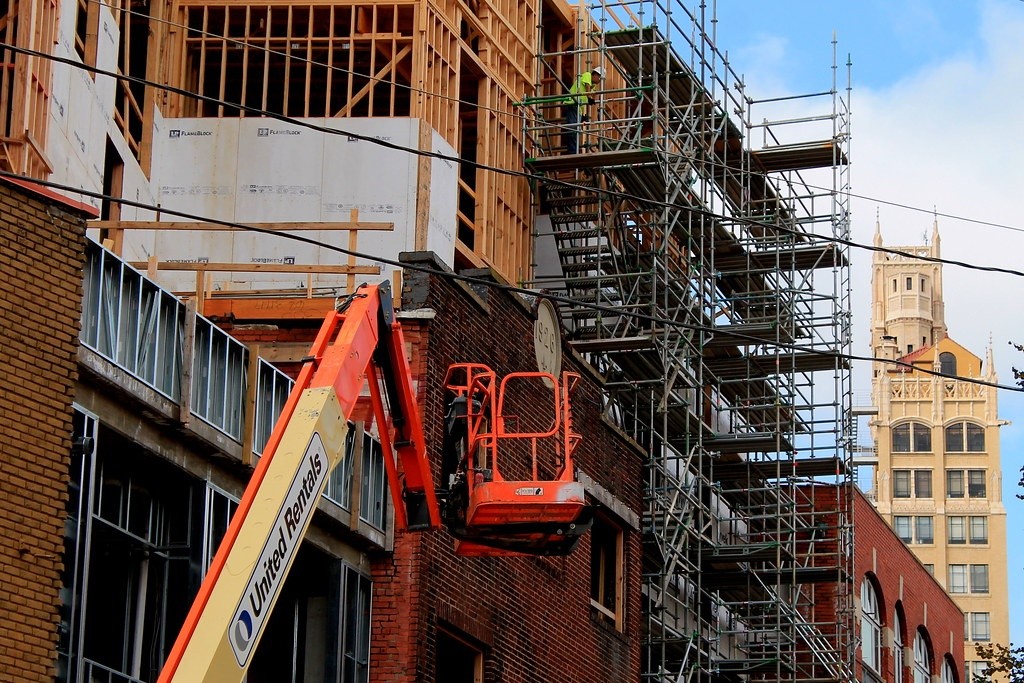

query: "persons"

left=563, top=66, right=607, bottom=155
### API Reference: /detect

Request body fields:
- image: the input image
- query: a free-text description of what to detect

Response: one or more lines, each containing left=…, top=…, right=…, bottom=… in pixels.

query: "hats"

left=592, top=66, right=606, bottom=78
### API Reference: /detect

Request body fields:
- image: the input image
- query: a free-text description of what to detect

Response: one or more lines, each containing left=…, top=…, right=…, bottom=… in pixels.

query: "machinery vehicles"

left=154, top=277, right=599, bottom=683
